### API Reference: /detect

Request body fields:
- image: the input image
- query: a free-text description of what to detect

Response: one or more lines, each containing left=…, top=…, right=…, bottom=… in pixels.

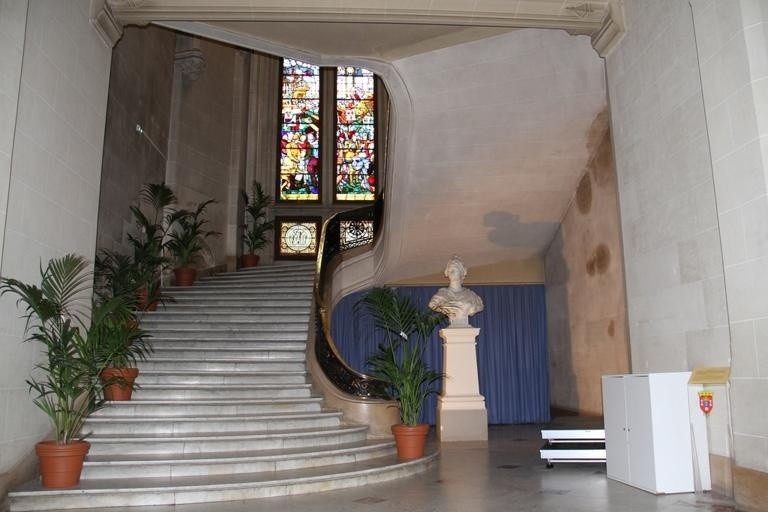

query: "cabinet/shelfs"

left=602, top=371, right=696, bottom=494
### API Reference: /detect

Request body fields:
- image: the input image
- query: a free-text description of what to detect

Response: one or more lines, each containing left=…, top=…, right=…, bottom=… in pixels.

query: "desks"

left=539, top=416, right=607, bottom=468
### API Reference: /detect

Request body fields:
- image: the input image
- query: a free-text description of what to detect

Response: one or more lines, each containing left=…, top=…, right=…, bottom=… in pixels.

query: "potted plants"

left=354, top=283, right=455, bottom=461
left=95, top=250, right=176, bottom=331
left=90, top=284, right=179, bottom=401
left=239, top=181, right=275, bottom=266
left=168, top=198, right=221, bottom=286
left=1, top=251, right=152, bottom=487
left=127, top=179, right=196, bottom=311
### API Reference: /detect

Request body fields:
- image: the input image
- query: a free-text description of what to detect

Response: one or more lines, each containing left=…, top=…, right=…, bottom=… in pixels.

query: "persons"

left=428, top=255, right=484, bottom=318
